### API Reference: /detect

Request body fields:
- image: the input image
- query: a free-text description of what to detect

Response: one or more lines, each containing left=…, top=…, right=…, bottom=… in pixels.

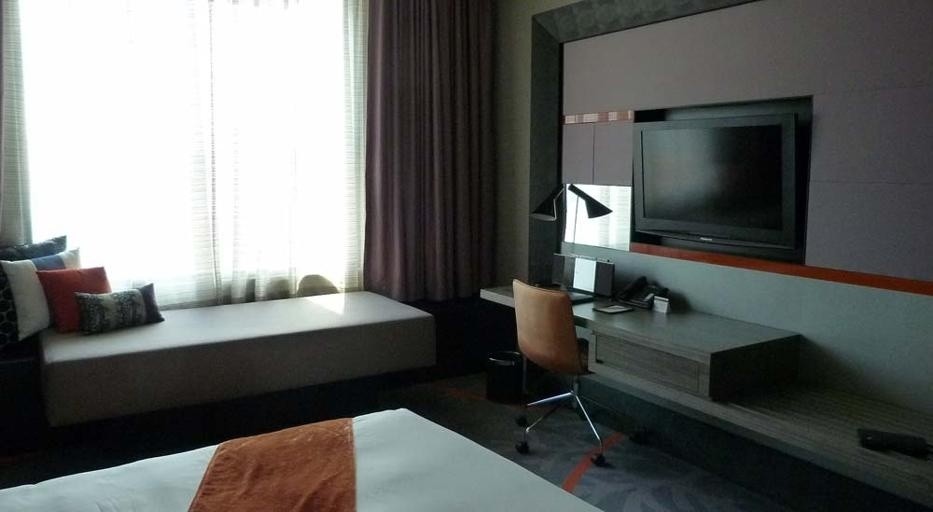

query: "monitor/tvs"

left=631, top=111, right=801, bottom=250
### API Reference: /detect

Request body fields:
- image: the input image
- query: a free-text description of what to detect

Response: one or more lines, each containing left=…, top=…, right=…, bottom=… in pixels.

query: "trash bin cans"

left=487, top=350, right=524, bottom=403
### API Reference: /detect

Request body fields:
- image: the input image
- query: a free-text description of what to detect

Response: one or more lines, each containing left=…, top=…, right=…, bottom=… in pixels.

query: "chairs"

left=508, top=280, right=648, bottom=467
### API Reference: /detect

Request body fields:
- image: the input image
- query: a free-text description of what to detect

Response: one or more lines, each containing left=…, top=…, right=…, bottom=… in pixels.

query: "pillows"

left=0, top=235, right=66, bottom=350
left=36, top=267, right=111, bottom=334
left=0, top=248, right=81, bottom=341
left=74, top=283, right=165, bottom=336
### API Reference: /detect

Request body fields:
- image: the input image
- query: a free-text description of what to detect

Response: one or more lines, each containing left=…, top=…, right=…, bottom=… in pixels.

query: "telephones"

left=616, top=276, right=670, bottom=310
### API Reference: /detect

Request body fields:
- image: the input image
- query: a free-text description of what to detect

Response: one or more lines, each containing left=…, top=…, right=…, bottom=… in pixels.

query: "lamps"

left=569, top=183, right=613, bottom=243
left=529, top=180, right=595, bottom=304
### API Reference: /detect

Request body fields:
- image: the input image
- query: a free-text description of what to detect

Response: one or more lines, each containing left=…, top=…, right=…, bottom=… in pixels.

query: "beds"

left=42, top=290, right=440, bottom=454
left=0, top=405, right=602, bottom=512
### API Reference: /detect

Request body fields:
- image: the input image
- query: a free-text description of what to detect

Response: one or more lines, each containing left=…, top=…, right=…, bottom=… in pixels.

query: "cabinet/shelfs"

left=480, top=282, right=933, bottom=512
